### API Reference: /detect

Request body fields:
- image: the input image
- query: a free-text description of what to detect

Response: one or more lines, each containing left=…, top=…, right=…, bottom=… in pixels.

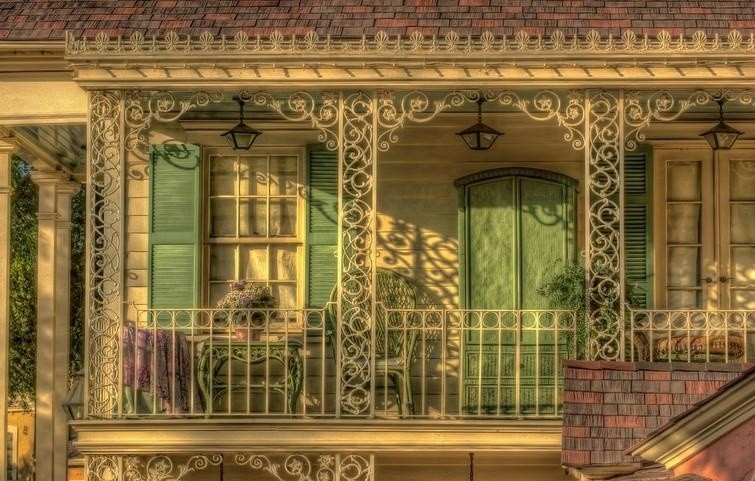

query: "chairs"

left=114, top=322, right=198, bottom=417
left=324, top=266, right=421, bottom=424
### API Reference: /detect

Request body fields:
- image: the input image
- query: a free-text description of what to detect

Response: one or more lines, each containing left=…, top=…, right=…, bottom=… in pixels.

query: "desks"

left=198, top=340, right=303, bottom=413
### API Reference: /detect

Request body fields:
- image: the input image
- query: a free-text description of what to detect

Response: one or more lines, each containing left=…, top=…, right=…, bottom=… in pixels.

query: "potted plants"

left=539, top=256, right=640, bottom=324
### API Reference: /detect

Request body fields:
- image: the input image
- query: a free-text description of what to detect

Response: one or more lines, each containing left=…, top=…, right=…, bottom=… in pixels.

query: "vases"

left=232, top=324, right=261, bottom=343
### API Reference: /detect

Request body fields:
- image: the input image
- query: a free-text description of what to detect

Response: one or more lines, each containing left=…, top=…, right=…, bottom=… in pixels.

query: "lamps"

left=456, top=99, right=504, bottom=152
left=60, top=367, right=84, bottom=420
left=220, top=97, right=261, bottom=152
left=700, top=97, right=746, bottom=154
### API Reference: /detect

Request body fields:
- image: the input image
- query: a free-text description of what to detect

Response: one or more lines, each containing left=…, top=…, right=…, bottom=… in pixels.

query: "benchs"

left=624, top=311, right=744, bottom=363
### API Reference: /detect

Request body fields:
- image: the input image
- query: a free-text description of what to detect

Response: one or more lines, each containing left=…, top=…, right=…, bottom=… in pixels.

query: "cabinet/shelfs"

left=451, top=166, right=581, bottom=421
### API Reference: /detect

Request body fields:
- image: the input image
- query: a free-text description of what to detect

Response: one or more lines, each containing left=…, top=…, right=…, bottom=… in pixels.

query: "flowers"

left=215, top=280, right=276, bottom=325
